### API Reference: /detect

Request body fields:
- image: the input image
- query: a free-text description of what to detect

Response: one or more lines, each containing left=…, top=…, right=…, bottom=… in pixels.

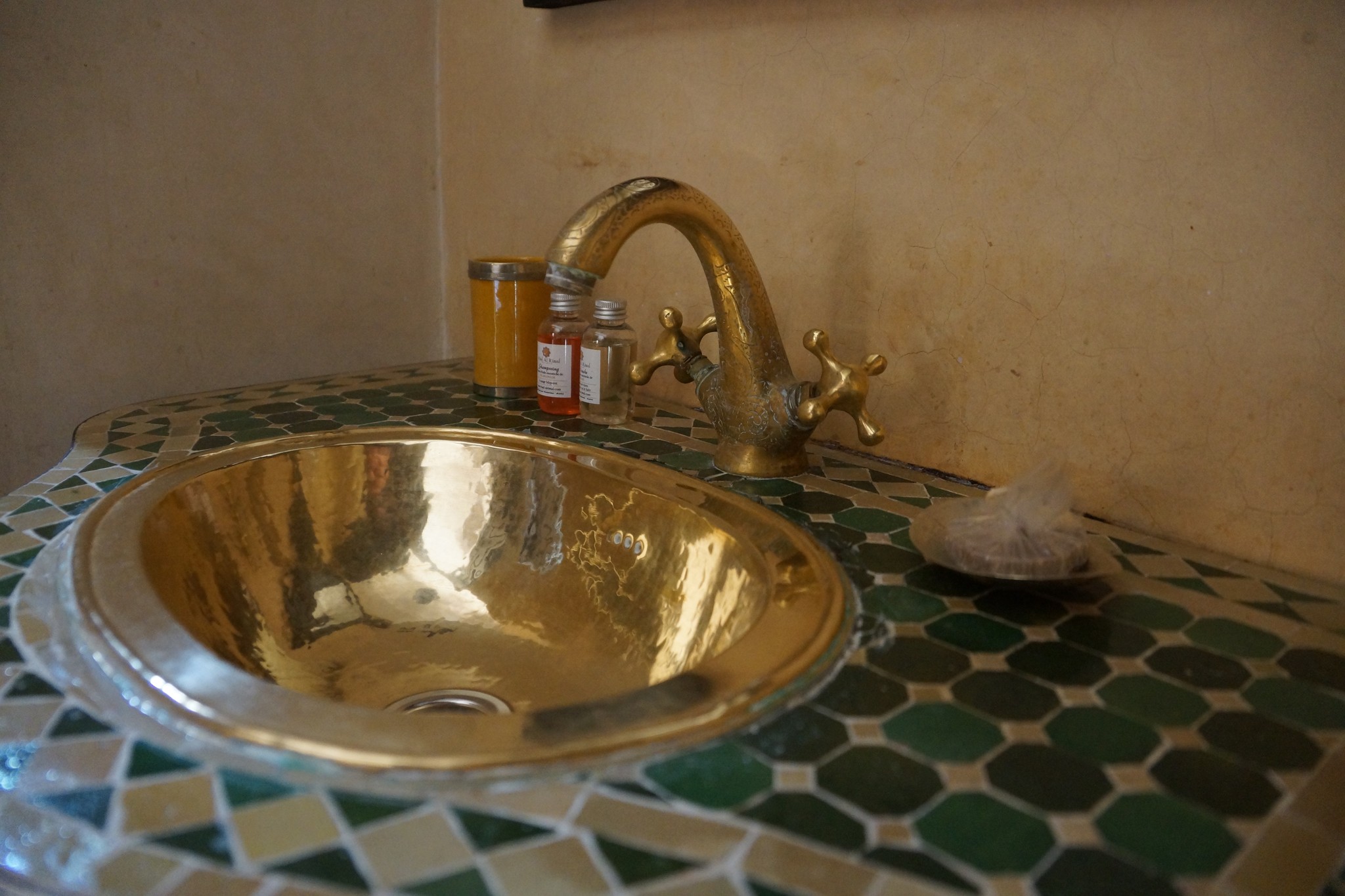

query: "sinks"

left=64, top=416, right=860, bottom=773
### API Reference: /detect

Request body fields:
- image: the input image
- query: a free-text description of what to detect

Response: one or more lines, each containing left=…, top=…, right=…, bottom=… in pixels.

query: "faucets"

left=544, top=169, right=890, bottom=482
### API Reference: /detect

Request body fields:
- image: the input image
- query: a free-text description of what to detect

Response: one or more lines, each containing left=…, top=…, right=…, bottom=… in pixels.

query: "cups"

left=467, top=254, right=553, bottom=398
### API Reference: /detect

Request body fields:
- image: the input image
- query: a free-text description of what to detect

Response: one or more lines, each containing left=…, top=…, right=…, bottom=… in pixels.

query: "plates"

left=909, top=496, right=1126, bottom=582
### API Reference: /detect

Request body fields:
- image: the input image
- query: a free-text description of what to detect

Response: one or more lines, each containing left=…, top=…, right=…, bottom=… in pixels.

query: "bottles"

left=537, top=289, right=588, bottom=415
left=578, top=299, right=637, bottom=426
